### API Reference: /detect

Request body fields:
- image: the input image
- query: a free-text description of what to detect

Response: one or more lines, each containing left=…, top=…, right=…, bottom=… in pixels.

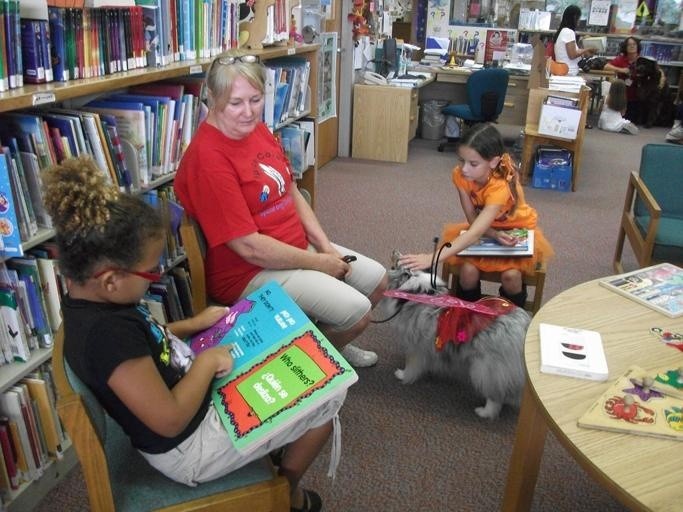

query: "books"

left=2, top=1, right=308, bottom=500
left=575, top=363, right=682, bottom=443
left=183, top=279, right=359, bottom=459
left=600, top=38, right=683, bottom=87
left=628, top=364, right=682, bottom=402
left=537, top=321, right=609, bottom=382
left=647, top=325, right=682, bottom=351
left=455, top=229, right=536, bottom=259
left=581, top=36, right=607, bottom=54
left=467, top=226, right=529, bottom=249
left=597, top=261, right=682, bottom=320
left=533, top=74, right=586, bottom=167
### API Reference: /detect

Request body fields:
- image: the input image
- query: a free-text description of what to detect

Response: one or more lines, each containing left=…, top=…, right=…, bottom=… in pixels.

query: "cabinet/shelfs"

left=351, top=65, right=528, bottom=163
left=0, top=0, right=321, bottom=510
left=532, top=31, right=683, bottom=117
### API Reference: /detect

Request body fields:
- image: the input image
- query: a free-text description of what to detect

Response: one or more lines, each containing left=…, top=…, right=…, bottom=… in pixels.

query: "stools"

left=442, top=261, right=546, bottom=316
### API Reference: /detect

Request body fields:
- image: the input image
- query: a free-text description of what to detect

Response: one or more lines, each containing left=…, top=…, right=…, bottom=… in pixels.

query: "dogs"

left=380, top=248, right=533, bottom=421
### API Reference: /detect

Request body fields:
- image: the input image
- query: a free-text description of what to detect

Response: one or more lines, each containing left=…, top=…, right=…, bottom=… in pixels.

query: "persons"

left=596, top=78, right=640, bottom=136
left=170, top=45, right=388, bottom=371
left=37, top=150, right=348, bottom=511
left=394, top=121, right=557, bottom=313
left=599, top=34, right=671, bottom=128
left=552, top=2, right=593, bottom=129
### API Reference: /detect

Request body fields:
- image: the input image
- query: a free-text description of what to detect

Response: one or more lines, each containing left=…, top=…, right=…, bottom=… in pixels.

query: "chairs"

left=52, top=320, right=291, bottom=512
left=611, top=144, right=683, bottom=273
left=180, top=216, right=208, bottom=316
left=438, top=69, right=509, bottom=153
left=521, top=85, right=592, bottom=191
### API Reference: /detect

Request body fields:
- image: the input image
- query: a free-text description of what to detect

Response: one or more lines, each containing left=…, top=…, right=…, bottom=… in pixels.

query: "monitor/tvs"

left=383, top=39, right=397, bottom=72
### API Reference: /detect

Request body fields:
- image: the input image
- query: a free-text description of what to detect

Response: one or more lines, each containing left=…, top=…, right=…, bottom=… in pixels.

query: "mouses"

left=417, top=75, right=426, bottom=80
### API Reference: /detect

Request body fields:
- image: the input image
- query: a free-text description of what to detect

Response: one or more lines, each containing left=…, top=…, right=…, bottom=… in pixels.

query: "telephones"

left=364, top=70, right=387, bottom=86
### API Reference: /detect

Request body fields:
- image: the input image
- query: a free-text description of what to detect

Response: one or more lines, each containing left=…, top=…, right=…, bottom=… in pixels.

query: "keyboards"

left=398, top=74, right=417, bottom=79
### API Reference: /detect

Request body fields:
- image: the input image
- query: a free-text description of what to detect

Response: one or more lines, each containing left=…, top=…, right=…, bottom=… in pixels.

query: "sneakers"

left=341, top=341, right=378, bottom=368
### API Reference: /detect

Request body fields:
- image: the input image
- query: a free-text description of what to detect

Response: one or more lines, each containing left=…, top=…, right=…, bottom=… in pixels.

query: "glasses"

left=210, top=53, right=260, bottom=71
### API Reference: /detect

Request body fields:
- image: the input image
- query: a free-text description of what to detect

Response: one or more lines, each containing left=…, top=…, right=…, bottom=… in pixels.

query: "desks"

left=499, top=270, right=683, bottom=512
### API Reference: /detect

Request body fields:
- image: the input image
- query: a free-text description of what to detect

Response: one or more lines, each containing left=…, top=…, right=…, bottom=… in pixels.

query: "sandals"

left=584, top=121, right=593, bottom=130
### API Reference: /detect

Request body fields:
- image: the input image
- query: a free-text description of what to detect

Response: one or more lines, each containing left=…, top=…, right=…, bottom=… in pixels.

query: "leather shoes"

left=289, top=488, right=323, bottom=512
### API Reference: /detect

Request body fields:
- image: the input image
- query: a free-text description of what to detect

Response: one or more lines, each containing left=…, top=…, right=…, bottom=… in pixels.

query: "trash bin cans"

left=420, top=99, right=449, bottom=140
